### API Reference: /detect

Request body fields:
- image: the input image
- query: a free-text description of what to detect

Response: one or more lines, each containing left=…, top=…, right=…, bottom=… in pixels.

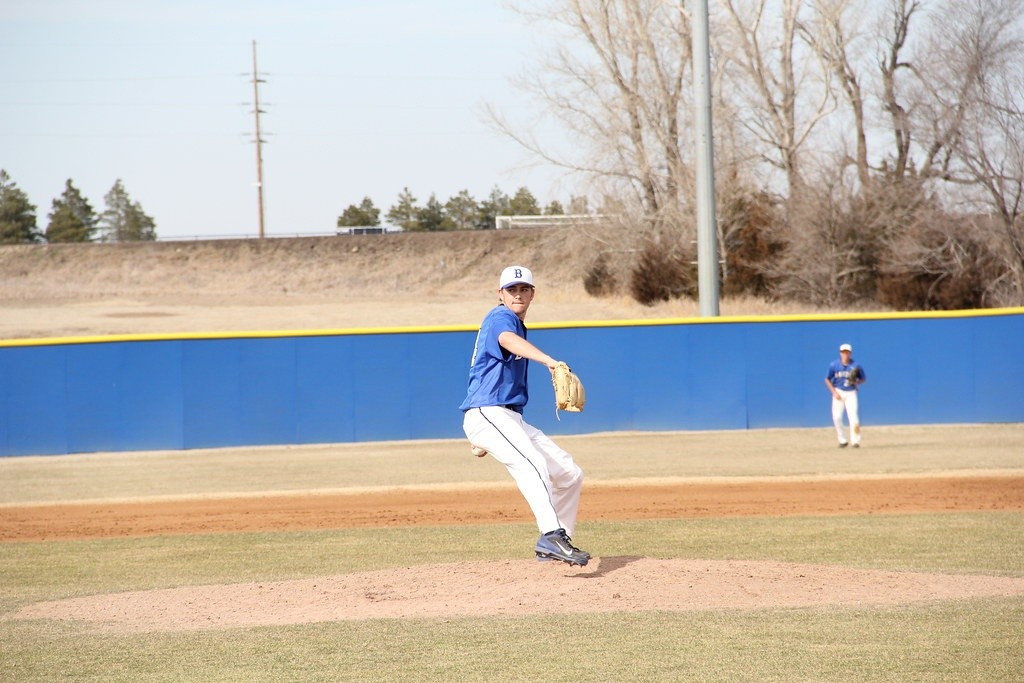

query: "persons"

left=460, top=265, right=590, bottom=567
left=825, top=343, right=866, bottom=449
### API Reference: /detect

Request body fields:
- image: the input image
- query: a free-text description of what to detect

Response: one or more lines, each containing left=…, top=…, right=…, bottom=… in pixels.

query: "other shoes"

left=853, top=443, right=859, bottom=449
left=839, top=442, right=849, bottom=447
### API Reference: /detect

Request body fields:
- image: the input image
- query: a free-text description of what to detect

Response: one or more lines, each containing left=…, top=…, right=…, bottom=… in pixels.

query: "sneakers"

left=535, top=527, right=590, bottom=565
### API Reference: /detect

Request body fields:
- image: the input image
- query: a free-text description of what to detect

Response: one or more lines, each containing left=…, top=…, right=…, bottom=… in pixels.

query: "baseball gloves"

left=550, top=360, right=586, bottom=422
left=847, top=367, right=858, bottom=385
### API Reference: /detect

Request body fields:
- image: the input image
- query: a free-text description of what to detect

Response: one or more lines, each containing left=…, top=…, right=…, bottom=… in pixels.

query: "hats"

left=839, top=344, right=853, bottom=353
left=498, top=265, right=535, bottom=290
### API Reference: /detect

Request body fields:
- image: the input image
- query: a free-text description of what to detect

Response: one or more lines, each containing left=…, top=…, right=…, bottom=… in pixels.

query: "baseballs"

left=471, top=445, right=485, bottom=456
